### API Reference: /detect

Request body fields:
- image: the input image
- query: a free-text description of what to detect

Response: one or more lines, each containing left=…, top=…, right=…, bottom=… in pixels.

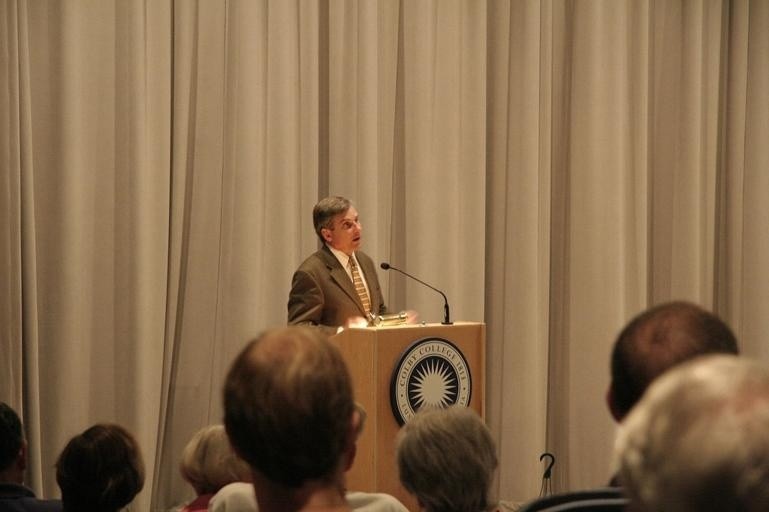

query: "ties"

left=349, top=257, right=371, bottom=312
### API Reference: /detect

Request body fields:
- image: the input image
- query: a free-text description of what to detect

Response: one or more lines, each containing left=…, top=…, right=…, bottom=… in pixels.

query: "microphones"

left=380, top=263, right=453, bottom=324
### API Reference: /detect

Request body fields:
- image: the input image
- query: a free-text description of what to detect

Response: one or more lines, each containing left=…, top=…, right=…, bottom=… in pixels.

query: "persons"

left=605, top=297, right=742, bottom=488
left=207, top=398, right=409, bottom=510
left=612, top=353, right=767, bottom=510
left=219, top=326, right=367, bottom=510
left=53, top=423, right=146, bottom=510
left=284, top=195, right=422, bottom=337
left=391, top=405, right=503, bottom=509
left=0, top=401, right=64, bottom=510
left=179, top=424, right=256, bottom=512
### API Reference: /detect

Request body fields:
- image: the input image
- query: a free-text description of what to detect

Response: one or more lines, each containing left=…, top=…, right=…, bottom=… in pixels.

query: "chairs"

left=520, top=486, right=631, bottom=511
